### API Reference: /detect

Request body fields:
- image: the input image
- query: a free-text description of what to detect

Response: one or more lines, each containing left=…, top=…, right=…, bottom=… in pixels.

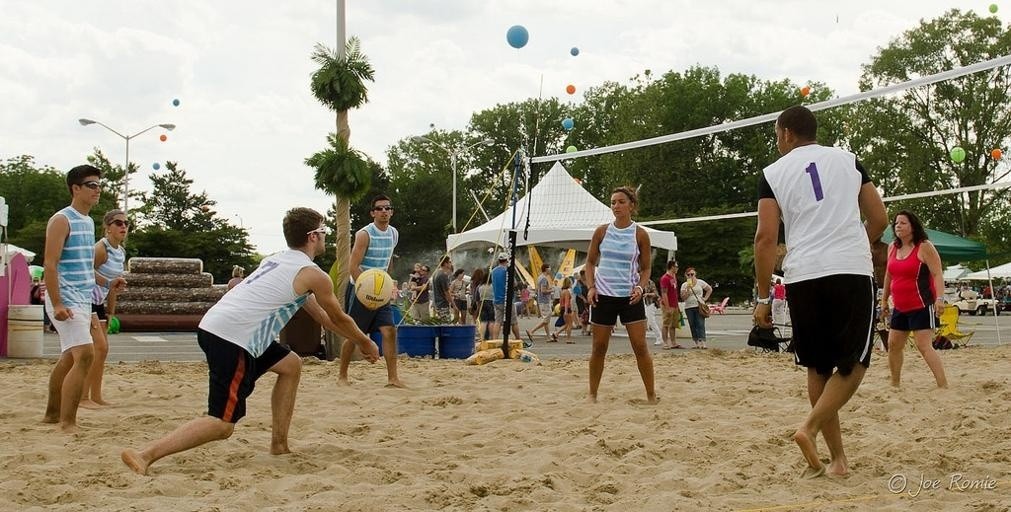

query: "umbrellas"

left=0, top=243, right=37, bottom=265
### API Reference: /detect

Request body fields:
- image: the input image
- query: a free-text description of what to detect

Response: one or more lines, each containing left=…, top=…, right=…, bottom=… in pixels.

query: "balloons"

left=171, top=97, right=180, bottom=106
left=565, top=82, right=576, bottom=95
left=990, top=148, right=1002, bottom=158
left=561, top=116, right=575, bottom=129
left=152, top=162, right=161, bottom=170
left=569, top=45, right=579, bottom=57
left=505, top=22, right=528, bottom=49
left=564, top=144, right=578, bottom=157
left=949, top=147, right=966, bottom=163
left=159, top=134, right=167, bottom=142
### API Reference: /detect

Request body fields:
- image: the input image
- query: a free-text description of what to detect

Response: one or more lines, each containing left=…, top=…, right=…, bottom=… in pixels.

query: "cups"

left=687, top=279, right=693, bottom=286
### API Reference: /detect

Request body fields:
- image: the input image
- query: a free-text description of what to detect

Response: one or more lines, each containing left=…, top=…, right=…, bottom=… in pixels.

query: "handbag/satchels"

left=696, top=301, right=712, bottom=318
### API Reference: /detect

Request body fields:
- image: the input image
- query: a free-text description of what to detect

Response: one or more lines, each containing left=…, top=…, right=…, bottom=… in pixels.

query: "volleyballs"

left=355, top=269, right=394, bottom=310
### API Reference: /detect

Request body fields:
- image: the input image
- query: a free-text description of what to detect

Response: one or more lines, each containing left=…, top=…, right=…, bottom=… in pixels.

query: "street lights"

left=404, top=131, right=496, bottom=237
left=77, top=117, right=177, bottom=259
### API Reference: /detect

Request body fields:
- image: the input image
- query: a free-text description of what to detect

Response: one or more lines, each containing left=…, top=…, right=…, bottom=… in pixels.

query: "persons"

left=30, top=283, right=55, bottom=335
left=76, top=208, right=130, bottom=409
left=883, top=209, right=948, bottom=393
left=752, top=105, right=889, bottom=478
left=120, top=208, right=380, bottom=479
left=584, top=186, right=660, bottom=404
left=338, top=194, right=413, bottom=391
left=228, top=263, right=245, bottom=291
left=40, top=164, right=102, bottom=436
left=35, top=271, right=46, bottom=305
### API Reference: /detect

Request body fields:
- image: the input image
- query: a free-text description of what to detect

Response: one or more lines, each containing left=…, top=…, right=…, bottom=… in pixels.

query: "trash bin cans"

left=280, top=307, right=327, bottom=360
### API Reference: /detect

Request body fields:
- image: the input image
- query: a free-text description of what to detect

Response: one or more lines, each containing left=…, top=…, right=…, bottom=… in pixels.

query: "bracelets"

left=935, top=296, right=944, bottom=301
left=634, top=285, right=644, bottom=294
left=755, top=296, right=770, bottom=305
left=588, top=286, right=595, bottom=292
left=103, top=277, right=111, bottom=289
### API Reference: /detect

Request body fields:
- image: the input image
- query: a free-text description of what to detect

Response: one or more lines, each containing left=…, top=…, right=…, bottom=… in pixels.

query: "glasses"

left=373, top=204, right=393, bottom=213
left=685, top=272, right=696, bottom=277
left=80, top=178, right=103, bottom=191
left=106, top=216, right=131, bottom=230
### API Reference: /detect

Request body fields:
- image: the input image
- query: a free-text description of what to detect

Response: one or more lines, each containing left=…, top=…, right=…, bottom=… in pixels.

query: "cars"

left=945, top=283, right=1001, bottom=318
left=395, top=273, right=473, bottom=309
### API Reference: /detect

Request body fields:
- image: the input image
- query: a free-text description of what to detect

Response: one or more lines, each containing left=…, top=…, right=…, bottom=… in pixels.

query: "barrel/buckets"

left=7, top=304, right=44, bottom=359
left=437, top=325, right=477, bottom=360
left=395, top=324, right=436, bottom=360
left=369, top=305, right=403, bottom=357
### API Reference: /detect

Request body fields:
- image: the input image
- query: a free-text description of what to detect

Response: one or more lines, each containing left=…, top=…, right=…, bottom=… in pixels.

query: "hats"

left=497, top=251, right=512, bottom=261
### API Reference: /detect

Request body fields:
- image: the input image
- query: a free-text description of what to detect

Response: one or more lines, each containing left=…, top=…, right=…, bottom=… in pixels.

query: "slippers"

left=525, top=327, right=535, bottom=342
left=661, top=344, right=686, bottom=351
left=545, top=331, right=559, bottom=344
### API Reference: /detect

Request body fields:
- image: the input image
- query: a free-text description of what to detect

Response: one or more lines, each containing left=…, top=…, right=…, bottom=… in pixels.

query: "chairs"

left=871, top=315, right=916, bottom=353
left=937, top=305, right=977, bottom=350
left=753, top=319, right=792, bottom=353
left=705, top=295, right=732, bottom=316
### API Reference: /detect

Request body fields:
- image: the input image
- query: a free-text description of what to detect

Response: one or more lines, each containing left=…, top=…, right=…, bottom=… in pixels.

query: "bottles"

left=884, top=306, right=892, bottom=332
